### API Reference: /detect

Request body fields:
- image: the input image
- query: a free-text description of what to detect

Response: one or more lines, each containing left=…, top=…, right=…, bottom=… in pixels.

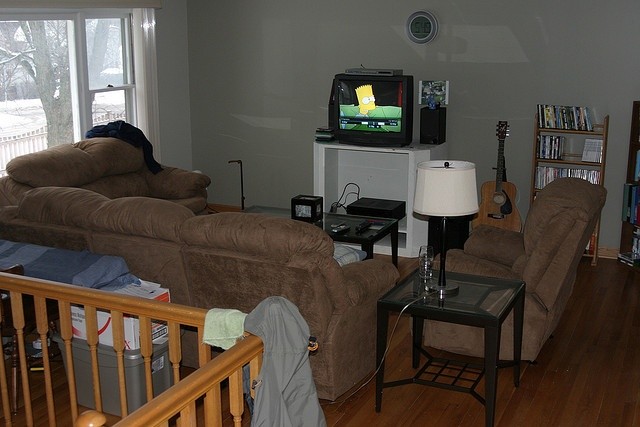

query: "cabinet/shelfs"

left=618, top=101, right=640, bottom=271
left=530, top=112, right=609, bottom=268
left=313, top=138, right=430, bottom=259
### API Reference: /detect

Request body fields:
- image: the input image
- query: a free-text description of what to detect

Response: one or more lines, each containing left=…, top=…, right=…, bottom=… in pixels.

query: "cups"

left=419, top=246, right=434, bottom=280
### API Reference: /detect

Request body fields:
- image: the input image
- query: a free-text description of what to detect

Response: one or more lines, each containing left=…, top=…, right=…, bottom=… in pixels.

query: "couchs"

left=409, top=177, right=607, bottom=363
left=0, top=136, right=401, bottom=402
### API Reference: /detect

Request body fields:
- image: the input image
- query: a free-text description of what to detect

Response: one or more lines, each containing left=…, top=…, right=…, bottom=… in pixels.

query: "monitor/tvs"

left=331, top=75, right=413, bottom=147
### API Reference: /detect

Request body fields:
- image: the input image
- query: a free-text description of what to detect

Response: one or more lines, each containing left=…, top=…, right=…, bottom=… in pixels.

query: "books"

left=535, top=165, right=603, bottom=195
left=581, top=138, right=603, bottom=163
left=618, top=151, right=640, bottom=265
left=536, top=135, right=565, bottom=162
left=536, top=104, right=593, bottom=133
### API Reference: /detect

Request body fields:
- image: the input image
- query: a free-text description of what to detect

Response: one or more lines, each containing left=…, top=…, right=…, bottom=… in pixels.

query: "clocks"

left=405, top=9, right=439, bottom=45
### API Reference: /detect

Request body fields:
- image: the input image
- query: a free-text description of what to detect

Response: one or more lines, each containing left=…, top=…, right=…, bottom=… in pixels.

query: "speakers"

left=420, top=107, right=446, bottom=145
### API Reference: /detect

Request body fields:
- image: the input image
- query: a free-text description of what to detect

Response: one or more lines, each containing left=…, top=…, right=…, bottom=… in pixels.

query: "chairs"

left=0, top=262, right=69, bottom=414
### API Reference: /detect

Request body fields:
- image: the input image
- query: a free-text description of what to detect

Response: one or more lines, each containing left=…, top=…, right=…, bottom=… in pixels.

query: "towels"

left=203, top=302, right=247, bottom=350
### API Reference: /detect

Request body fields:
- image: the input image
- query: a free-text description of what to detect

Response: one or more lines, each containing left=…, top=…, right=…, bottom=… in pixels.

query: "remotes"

left=331, top=225, right=351, bottom=233
left=331, top=220, right=344, bottom=228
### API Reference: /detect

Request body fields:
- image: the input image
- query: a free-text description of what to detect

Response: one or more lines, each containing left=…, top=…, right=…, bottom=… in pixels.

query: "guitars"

left=471, top=120, right=522, bottom=233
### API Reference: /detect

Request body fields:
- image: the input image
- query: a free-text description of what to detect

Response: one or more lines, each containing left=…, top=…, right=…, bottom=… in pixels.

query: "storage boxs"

left=49, top=328, right=187, bottom=418
left=65, top=277, right=172, bottom=350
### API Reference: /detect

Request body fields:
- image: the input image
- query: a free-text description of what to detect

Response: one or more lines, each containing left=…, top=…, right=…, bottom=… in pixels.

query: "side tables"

left=375, top=267, right=526, bottom=427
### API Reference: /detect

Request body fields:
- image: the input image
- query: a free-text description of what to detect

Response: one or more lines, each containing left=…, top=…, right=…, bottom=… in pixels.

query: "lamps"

left=412, top=159, right=480, bottom=296
